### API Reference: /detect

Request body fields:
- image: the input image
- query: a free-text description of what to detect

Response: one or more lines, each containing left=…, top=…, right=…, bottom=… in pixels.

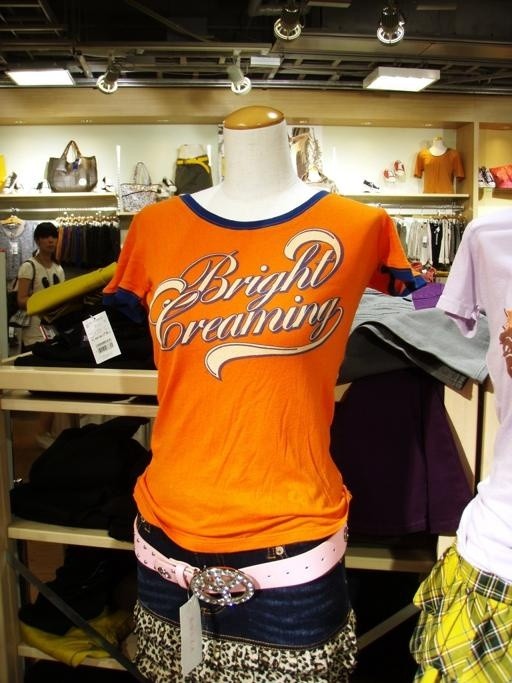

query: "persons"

left=104, top=106, right=432, bottom=683
left=413, top=135, right=466, bottom=195
left=412, top=199, right=512, bottom=683
left=173, top=142, right=215, bottom=194
left=4, top=221, right=68, bottom=351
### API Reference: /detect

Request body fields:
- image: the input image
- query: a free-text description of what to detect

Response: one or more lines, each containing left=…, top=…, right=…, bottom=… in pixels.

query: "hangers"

left=50, top=205, right=123, bottom=231
left=1, top=208, right=34, bottom=230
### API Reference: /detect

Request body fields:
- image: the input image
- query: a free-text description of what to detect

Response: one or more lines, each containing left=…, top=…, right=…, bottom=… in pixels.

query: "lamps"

left=226, top=63, right=254, bottom=94
left=362, top=64, right=442, bottom=92
left=5, top=57, right=80, bottom=87
left=375, top=4, right=407, bottom=45
left=273, top=7, right=304, bottom=41
left=96, top=58, right=121, bottom=94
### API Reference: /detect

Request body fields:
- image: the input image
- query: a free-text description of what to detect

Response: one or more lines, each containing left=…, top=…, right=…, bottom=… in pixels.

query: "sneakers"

left=394, top=161, right=405, bottom=176
left=384, top=170, right=395, bottom=182
left=480, top=166, right=495, bottom=186
left=364, top=178, right=380, bottom=190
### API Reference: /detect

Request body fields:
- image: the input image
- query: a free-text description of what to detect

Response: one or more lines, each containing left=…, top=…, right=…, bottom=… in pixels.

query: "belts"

left=177, top=157, right=210, bottom=175
left=133, top=514, right=349, bottom=607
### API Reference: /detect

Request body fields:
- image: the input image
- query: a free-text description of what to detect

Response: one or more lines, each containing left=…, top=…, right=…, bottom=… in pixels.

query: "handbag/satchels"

left=491, top=165, right=512, bottom=190
left=8, top=290, right=31, bottom=327
left=121, top=162, right=160, bottom=211
left=46, top=141, right=97, bottom=191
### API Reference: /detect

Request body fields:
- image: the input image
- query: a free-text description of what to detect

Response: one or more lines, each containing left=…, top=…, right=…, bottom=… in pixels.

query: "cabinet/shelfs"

left=0, top=367, right=484, bottom=683
